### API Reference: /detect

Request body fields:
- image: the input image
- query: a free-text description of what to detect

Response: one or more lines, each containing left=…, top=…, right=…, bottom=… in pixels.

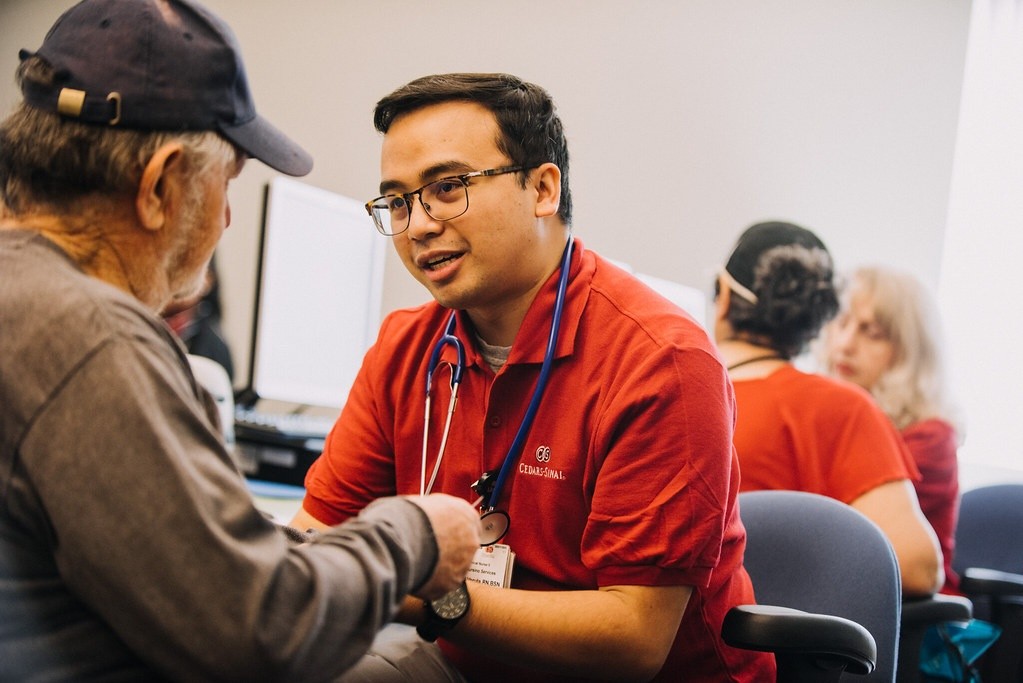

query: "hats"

left=16, top=0, right=313, bottom=178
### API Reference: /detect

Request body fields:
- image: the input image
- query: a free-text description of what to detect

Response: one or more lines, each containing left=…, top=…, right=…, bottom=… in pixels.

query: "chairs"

left=720, top=483, right=1023, bottom=683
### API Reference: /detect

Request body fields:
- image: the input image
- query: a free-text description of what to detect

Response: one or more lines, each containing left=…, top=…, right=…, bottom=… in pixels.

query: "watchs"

left=416, top=582, right=470, bottom=642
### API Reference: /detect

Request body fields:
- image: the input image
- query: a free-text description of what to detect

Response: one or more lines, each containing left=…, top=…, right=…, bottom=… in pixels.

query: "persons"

left=160, top=250, right=235, bottom=384
left=0, top=0, right=483, bottom=683
left=709, top=220, right=944, bottom=596
left=825, top=267, right=960, bottom=598
left=285, top=72, right=777, bottom=683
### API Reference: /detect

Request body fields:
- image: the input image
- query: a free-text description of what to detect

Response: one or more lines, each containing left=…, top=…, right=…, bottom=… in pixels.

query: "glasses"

left=364, top=163, right=542, bottom=237
